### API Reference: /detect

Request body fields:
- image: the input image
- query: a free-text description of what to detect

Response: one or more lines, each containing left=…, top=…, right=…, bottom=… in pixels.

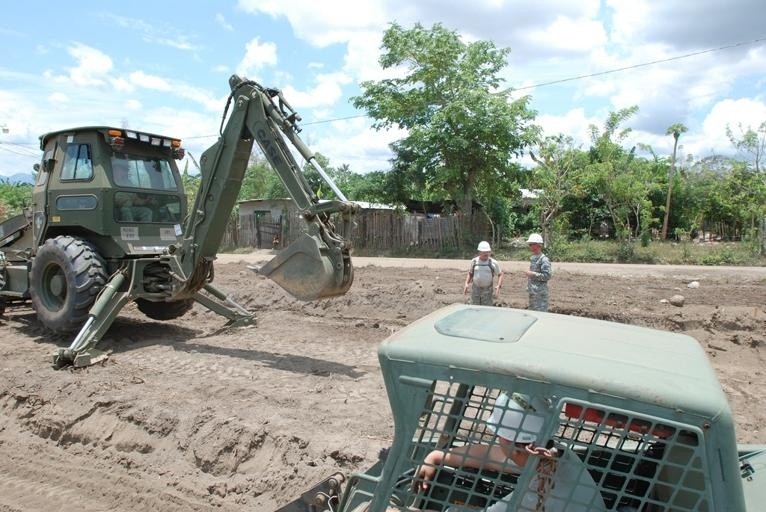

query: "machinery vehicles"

left=0, top=73, right=364, bottom=373
left=255, top=303, right=765, bottom=511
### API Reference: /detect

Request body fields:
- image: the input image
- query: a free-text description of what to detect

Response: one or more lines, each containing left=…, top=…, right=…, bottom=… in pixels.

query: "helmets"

left=112, top=158, right=132, bottom=170
left=526, top=233, right=543, bottom=245
left=486, top=392, right=560, bottom=444
left=477, top=241, right=491, bottom=252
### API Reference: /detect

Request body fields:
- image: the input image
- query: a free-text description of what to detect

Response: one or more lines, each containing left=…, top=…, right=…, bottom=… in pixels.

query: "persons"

left=464, top=241, right=504, bottom=305
left=113, top=163, right=154, bottom=221
left=525, top=233, right=552, bottom=312
left=410, top=391, right=606, bottom=512
